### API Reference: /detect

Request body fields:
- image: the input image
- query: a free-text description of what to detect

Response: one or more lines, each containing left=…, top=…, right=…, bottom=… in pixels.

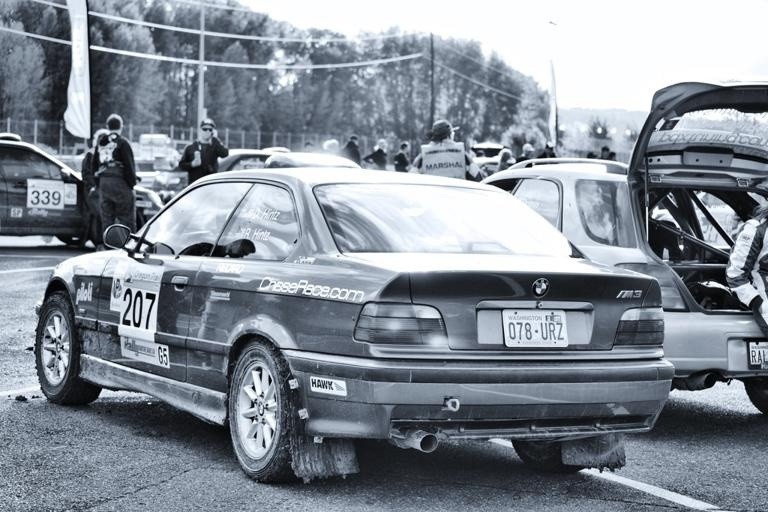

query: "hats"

left=431, top=120, right=460, bottom=134
left=201, top=119, right=216, bottom=127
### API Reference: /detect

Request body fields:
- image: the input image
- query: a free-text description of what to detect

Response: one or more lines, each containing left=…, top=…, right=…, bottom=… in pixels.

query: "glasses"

left=202, top=128, right=212, bottom=131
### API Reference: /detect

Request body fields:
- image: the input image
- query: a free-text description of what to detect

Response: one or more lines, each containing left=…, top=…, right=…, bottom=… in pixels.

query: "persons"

left=178, top=116, right=229, bottom=185
left=90, top=112, right=136, bottom=232
left=414, top=120, right=483, bottom=182
left=177, top=238, right=256, bottom=258
left=724, top=200, right=767, bottom=338
left=466, top=142, right=617, bottom=173
left=80, top=128, right=108, bottom=245
left=302, top=135, right=421, bottom=171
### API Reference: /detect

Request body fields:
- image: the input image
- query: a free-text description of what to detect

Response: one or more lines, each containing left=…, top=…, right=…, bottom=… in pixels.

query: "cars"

left=474, top=75, right=766, bottom=422
left=0, top=126, right=165, bottom=246
left=28, top=166, right=677, bottom=489
left=81, top=138, right=361, bottom=206
